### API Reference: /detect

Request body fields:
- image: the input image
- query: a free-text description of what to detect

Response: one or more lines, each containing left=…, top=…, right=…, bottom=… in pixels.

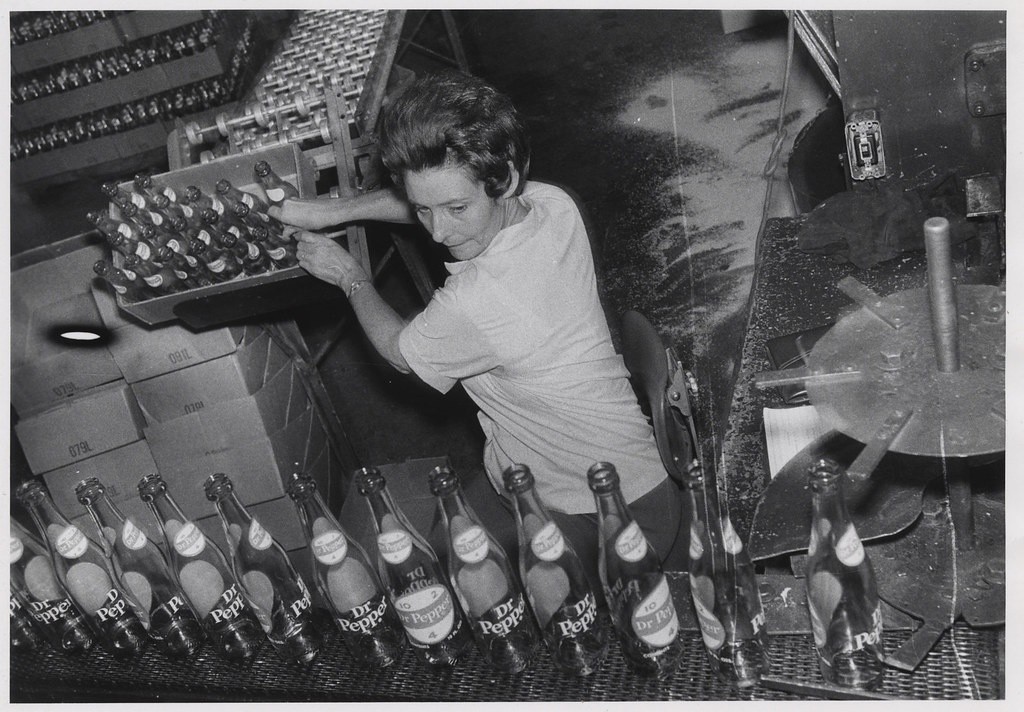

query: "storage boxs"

left=11, top=139, right=319, bottom=549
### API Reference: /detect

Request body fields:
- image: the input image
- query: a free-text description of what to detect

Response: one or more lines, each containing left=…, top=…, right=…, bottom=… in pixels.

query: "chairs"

left=446, top=312, right=717, bottom=631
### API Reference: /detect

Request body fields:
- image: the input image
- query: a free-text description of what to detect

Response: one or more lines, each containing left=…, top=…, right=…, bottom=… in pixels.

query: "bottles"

left=138, top=474, right=264, bottom=665
left=284, top=472, right=405, bottom=670
left=204, top=473, right=321, bottom=667
left=75, top=477, right=202, bottom=665
left=501, top=463, right=607, bottom=676
left=683, top=459, right=770, bottom=686
left=85, top=160, right=299, bottom=303
left=587, top=461, right=682, bottom=680
left=11, top=10, right=255, bottom=161
left=11, top=517, right=94, bottom=655
left=428, top=465, right=534, bottom=674
left=10, top=591, right=43, bottom=652
left=15, top=481, right=148, bottom=658
left=806, top=459, right=884, bottom=690
left=354, top=467, right=469, bottom=665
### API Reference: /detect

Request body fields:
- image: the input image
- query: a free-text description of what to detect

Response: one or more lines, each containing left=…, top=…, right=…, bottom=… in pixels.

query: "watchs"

left=347, top=281, right=368, bottom=301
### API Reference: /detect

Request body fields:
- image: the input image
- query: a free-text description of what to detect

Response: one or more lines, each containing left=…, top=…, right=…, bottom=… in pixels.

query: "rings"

left=301, top=253, right=305, bottom=259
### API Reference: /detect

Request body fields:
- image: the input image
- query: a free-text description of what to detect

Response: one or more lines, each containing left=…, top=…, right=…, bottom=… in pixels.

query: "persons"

left=269, top=70, right=682, bottom=603
